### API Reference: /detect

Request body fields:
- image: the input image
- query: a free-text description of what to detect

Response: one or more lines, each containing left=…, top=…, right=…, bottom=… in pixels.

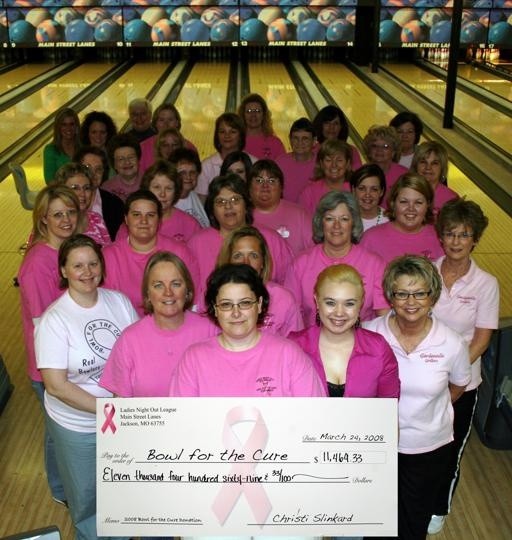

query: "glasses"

left=392, top=290, right=433, bottom=300
left=85, top=165, right=105, bottom=174
left=441, top=230, right=475, bottom=238
left=214, top=300, right=258, bottom=312
left=213, top=197, right=245, bottom=205
left=369, top=143, right=393, bottom=150
left=254, top=177, right=279, bottom=185
left=245, top=108, right=265, bottom=113
left=68, top=183, right=93, bottom=192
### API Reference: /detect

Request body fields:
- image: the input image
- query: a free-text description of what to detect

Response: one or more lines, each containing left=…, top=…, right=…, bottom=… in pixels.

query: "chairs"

left=6, top=160, right=43, bottom=254
